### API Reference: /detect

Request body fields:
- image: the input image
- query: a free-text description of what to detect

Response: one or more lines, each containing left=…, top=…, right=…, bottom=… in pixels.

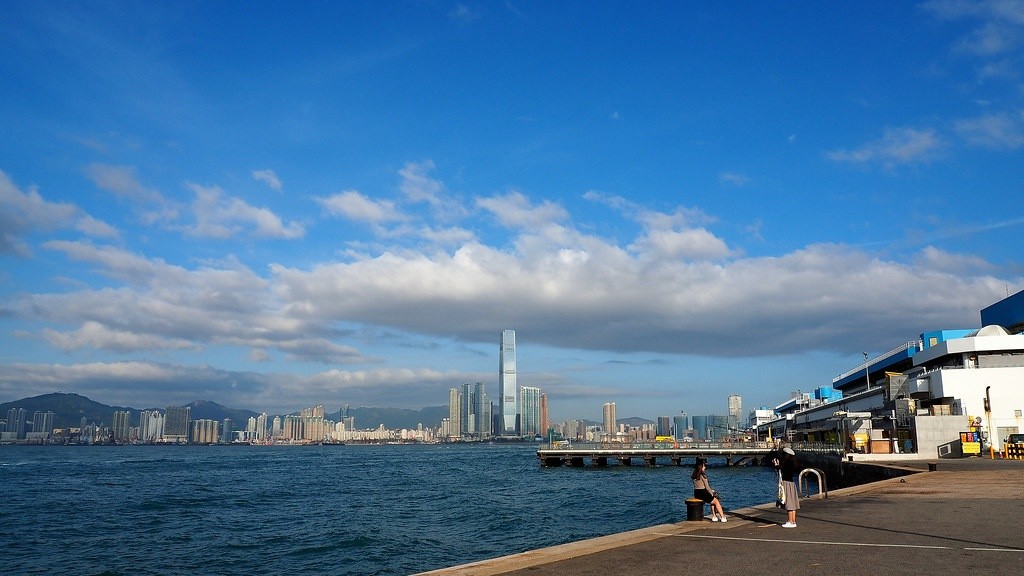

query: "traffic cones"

left=997, top=448, right=1004, bottom=459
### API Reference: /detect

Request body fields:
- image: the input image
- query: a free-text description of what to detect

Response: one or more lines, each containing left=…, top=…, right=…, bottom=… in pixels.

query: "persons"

left=691, top=462, right=727, bottom=522
left=772, top=447, right=800, bottom=528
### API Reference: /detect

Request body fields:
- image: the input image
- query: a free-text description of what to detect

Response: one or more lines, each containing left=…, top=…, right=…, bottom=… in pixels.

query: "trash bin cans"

left=903, top=440, right=913, bottom=453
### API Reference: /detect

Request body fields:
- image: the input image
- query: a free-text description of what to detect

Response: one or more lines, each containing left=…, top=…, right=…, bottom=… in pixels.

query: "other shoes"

left=782, top=522, right=797, bottom=528
left=721, top=517, right=727, bottom=523
left=712, top=517, right=721, bottom=522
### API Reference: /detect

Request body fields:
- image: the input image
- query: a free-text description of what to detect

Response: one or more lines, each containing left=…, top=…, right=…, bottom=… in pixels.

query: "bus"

left=655, top=436, right=675, bottom=443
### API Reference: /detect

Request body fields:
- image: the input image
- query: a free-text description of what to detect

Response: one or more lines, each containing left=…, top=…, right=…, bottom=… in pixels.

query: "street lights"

left=863, top=351, right=870, bottom=390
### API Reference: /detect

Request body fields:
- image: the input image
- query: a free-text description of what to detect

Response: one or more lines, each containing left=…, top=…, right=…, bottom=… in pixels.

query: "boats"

left=322, top=433, right=346, bottom=445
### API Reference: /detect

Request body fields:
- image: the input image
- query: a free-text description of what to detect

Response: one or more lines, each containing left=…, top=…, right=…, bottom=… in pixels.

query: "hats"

left=783, top=448, right=795, bottom=456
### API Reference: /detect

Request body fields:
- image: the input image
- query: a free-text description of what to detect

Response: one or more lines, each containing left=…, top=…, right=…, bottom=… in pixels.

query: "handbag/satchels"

left=712, top=492, right=722, bottom=500
left=778, top=468, right=785, bottom=504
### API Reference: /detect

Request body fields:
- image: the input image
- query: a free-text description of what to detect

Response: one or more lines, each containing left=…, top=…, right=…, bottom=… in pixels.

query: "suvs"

left=1004, top=434, right=1024, bottom=444
